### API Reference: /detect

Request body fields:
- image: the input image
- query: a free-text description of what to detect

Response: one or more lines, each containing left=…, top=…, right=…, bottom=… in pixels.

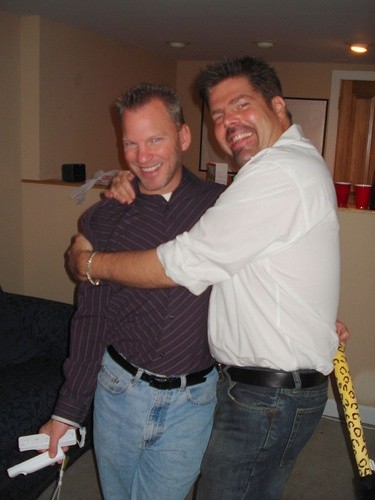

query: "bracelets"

left=86, top=251, right=101, bottom=287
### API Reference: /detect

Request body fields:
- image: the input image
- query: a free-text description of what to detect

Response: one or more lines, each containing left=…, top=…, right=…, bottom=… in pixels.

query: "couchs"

left=0, top=288, right=99, bottom=499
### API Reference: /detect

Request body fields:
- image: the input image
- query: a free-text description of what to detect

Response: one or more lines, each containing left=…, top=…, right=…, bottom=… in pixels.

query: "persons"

left=63, top=56, right=341, bottom=500
left=38, top=83, right=351, bottom=500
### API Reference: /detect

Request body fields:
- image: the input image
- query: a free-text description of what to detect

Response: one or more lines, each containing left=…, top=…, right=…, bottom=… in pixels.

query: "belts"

left=216, top=363, right=328, bottom=388
left=106, top=345, right=216, bottom=390
